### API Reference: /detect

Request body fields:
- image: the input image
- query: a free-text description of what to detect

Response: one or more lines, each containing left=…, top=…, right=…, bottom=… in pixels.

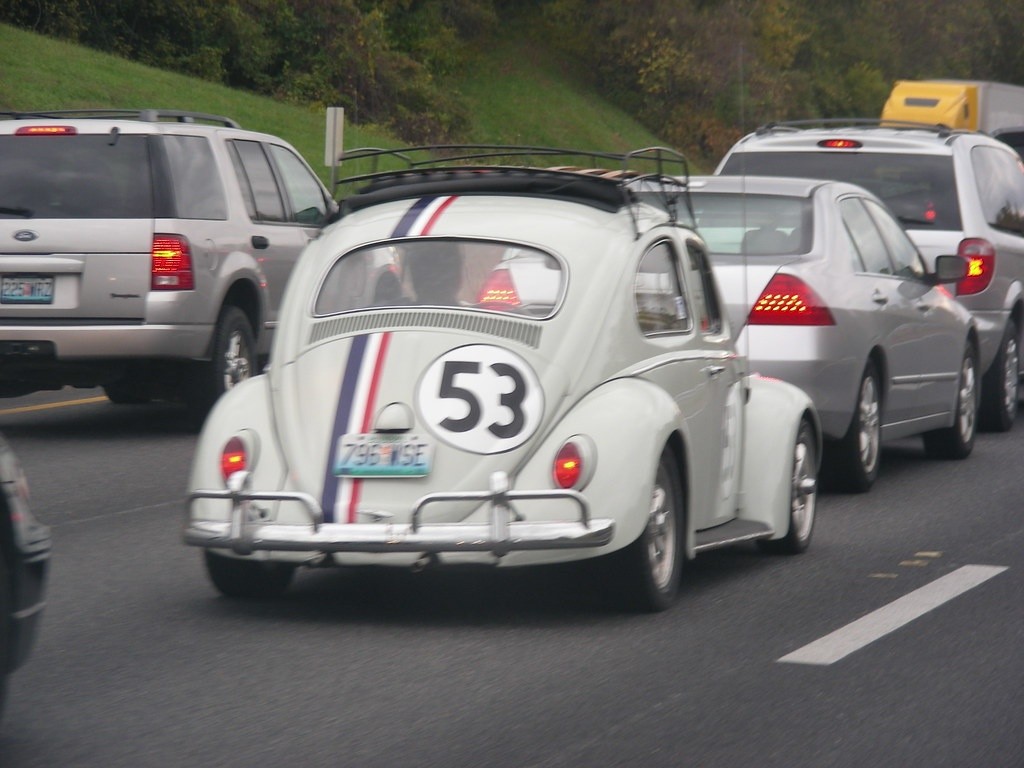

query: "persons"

left=406, top=237, right=467, bottom=306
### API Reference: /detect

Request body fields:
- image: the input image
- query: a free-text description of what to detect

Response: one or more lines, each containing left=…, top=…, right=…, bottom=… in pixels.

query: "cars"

left=185, top=144, right=820, bottom=611
left=477, top=174, right=982, bottom=496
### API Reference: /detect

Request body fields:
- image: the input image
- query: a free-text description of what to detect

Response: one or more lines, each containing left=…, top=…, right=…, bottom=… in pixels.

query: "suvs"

left=0, top=108, right=344, bottom=423
left=695, top=118, right=1024, bottom=439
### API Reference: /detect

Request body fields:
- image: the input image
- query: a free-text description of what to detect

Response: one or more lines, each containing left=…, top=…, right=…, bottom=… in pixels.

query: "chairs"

left=741, top=230, right=788, bottom=255
